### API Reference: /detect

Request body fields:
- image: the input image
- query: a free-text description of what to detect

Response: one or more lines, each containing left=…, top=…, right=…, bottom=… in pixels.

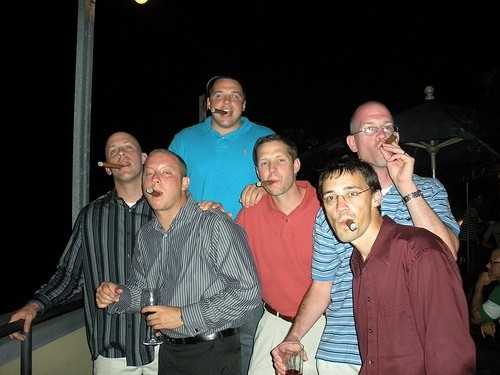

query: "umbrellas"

left=299, top=86, right=500, bottom=179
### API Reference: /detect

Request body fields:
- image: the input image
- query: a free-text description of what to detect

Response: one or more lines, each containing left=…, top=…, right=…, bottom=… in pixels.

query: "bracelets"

left=402, top=189, right=423, bottom=205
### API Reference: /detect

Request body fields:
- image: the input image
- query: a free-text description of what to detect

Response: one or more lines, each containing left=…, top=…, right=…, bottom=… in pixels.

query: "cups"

left=277, top=341, right=304, bottom=375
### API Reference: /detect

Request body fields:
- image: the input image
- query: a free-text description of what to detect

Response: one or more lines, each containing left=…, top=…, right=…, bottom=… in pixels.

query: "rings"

left=404, top=151, right=407, bottom=155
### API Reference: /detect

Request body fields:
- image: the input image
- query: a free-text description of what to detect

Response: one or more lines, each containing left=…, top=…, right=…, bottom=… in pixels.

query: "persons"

left=457, top=156, right=500, bottom=338
left=96, top=149, right=263, bottom=375
left=7, top=128, right=160, bottom=375
left=196, top=134, right=326, bottom=375
left=269, top=100, right=461, bottom=374
left=317, top=156, right=476, bottom=374
left=167, top=72, right=276, bottom=221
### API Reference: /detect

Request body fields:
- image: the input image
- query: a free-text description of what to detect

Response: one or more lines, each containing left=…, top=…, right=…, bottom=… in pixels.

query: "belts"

left=259, top=298, right=325, bottom=324
left=160, top=326, right=240, bottom=346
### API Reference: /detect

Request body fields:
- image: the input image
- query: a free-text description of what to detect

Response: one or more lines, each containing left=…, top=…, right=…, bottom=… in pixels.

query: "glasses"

left=321, top=188, right=371, bottom=205
left=351, top=125, right=399, bottom=136
left=489, top=259, right=500, bottom=265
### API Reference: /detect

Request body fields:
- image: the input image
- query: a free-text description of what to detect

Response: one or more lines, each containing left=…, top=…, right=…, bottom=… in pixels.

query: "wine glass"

left=140, top=287, right=164, bottom=346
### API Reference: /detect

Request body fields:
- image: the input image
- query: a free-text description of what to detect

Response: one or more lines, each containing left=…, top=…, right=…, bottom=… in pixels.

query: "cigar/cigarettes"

left=346, top=219, right=357, bottom=231
left=97, top=161, right=122, bottom=169
left=385, top=132, right=398, bottom=145
left=257, top=180, right=273, bottom=187
left=211, top=108, right=228, bottom=115
left=146, top=188, right=160, bottom=197
